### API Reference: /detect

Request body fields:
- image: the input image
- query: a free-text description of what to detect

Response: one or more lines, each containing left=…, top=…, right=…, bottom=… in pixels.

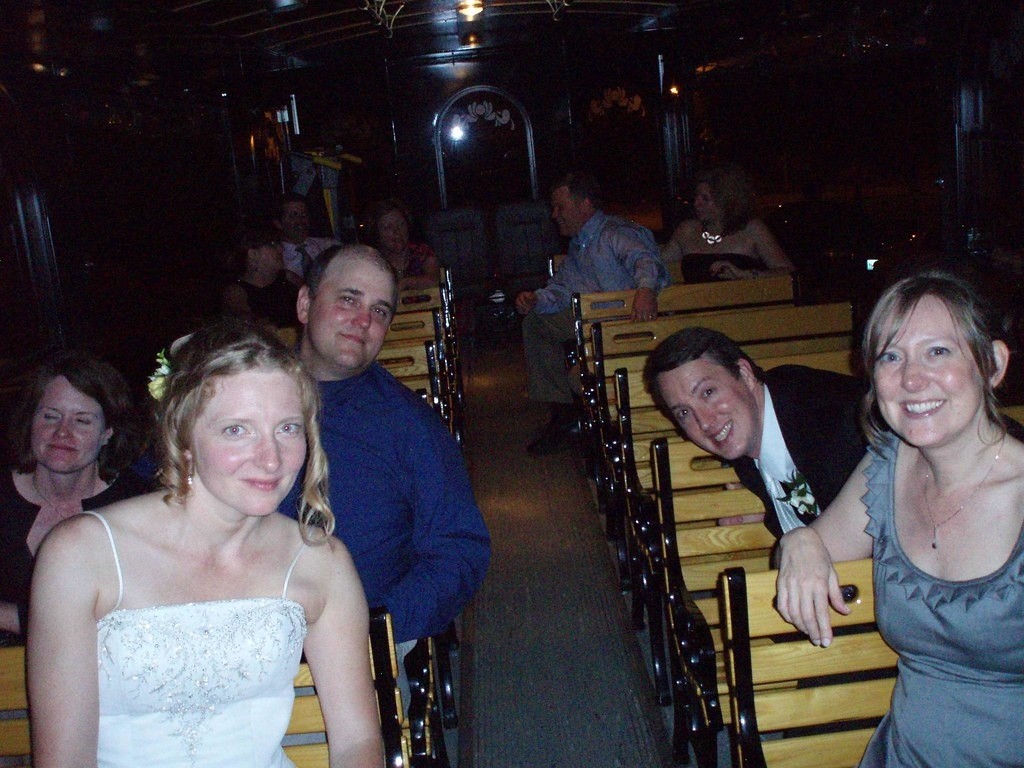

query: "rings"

left=648, top=315, right=653, bottom=319
left=642, top=316, right=647, bottom=319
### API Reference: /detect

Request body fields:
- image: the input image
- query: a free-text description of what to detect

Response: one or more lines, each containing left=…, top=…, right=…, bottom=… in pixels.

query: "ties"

left=293, top=244, right=315, bottom=286
left=759, top=464, right=806, bottom=534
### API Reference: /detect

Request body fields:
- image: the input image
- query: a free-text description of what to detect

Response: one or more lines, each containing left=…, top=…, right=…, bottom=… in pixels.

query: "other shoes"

left=527, top=400, right=586, bottom=455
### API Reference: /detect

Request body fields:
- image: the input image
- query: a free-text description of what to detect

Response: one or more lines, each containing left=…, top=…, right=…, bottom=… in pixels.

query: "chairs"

left=493, top=201, right=562, bottom=275
left=421, top=201, right=496, bottom=312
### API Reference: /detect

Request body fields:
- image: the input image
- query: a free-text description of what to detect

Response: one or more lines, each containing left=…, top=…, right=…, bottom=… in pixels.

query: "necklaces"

left=32, top=474, right=66, bottom=520
left=924, top=444, right=1005, bottom=549
left=702, top=221, right=727, bottom=244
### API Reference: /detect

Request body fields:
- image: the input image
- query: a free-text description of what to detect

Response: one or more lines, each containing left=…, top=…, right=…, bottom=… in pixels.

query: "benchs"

left=550, top=251, right=1024, bottom=768
left=0, top=265, right=459, bottom=768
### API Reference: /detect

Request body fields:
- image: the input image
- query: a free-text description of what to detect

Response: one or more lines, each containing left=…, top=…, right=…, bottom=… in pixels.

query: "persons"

left=636, top=168, right=796, bottom=285
left=135, top=242, right=495, bottom=715
left=0, top=352, right=150, bottom=635
left=515, top=172, right=672, bottom=455
left=773, top=269, right=1024, bottom=767
left=28, top=322, right=385, bottom=768
left=221, top=193, right=438, bottom=330
left=641, top=328, right=869, bottom=536
